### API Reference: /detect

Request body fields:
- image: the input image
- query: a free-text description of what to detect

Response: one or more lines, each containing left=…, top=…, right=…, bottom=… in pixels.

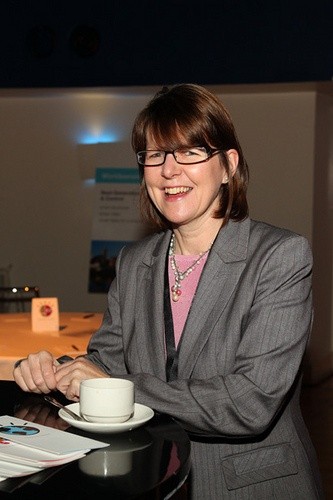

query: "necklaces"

left=169, top=232, right=211, bottom=302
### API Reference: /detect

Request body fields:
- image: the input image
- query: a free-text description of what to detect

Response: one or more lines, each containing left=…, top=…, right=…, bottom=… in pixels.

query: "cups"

left=79, top=378, right=134, bottom=423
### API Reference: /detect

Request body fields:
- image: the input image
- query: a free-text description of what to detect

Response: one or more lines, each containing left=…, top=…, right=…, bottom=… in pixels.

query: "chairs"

left=0, top=286, right=41, bottom=314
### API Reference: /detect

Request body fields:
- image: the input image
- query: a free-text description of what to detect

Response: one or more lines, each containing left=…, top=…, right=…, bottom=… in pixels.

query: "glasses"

left=136, top=146, right=225, bottom=166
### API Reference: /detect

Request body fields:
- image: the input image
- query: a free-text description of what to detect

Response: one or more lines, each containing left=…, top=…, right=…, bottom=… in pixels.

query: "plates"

left=58, top=402, right=154, bottom=432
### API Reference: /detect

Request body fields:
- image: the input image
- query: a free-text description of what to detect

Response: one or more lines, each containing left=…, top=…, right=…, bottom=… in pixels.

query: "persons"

left=13, top=84, right=323, bottom=500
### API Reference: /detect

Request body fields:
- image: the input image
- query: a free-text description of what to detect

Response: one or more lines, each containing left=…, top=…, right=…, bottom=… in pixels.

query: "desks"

left=0, top=379, right=192, bottom=500
left=0, top=311, right=105, bottom=383
